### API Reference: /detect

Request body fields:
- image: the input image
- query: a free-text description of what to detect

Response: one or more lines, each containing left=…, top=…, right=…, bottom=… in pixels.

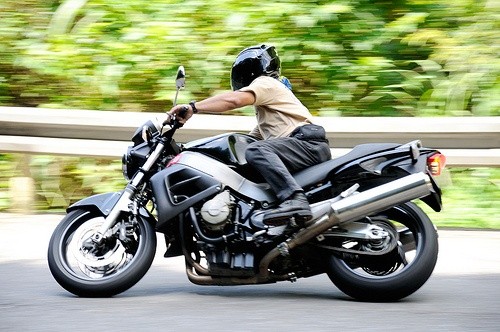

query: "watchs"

left=189, top=101, right=199, bottom=113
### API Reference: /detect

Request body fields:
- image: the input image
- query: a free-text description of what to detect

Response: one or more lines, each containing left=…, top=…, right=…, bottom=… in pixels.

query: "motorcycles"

left=46, top=65, right=446, bottom=304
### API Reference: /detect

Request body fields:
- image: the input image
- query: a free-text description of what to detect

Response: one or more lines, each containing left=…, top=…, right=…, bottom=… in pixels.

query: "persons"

left=166, top=43, right=331, bottom=225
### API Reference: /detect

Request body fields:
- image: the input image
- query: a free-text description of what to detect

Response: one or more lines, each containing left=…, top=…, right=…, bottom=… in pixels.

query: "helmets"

left=229, top=44, right=282, bottom=91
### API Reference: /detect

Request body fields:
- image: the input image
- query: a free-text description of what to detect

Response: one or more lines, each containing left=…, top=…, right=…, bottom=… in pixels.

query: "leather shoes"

left=262, top=191, right=313, bottom=227
